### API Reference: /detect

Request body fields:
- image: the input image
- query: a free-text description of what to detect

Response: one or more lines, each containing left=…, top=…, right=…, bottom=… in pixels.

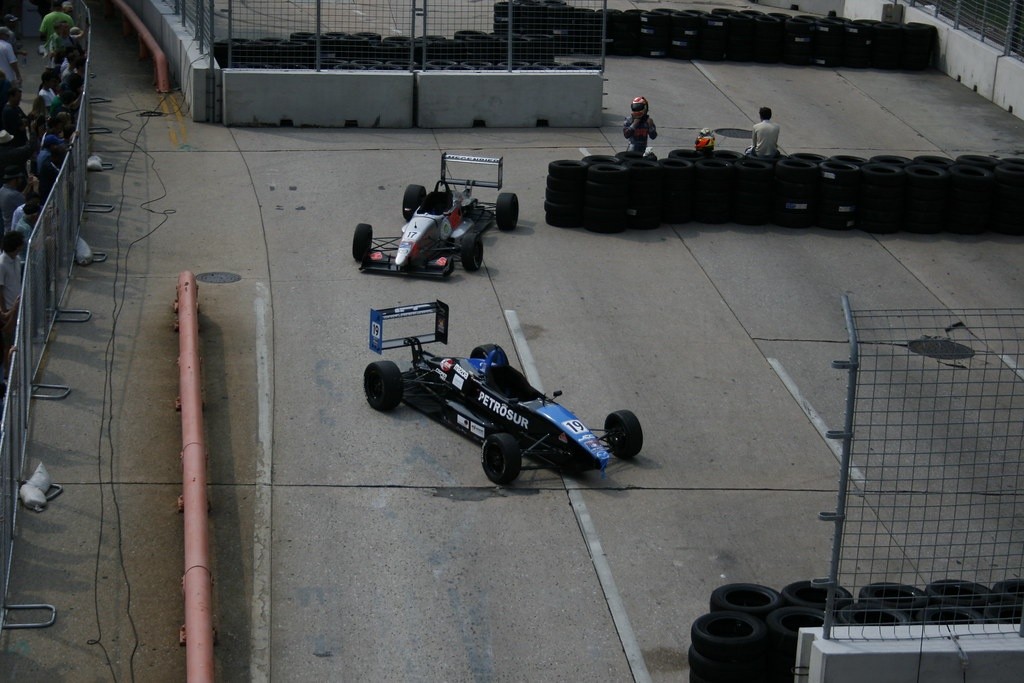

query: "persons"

left=1, top=0, right=85, bottom=420
left=695, top=128, right=716, bottom=151
left=623, top=96, right=658, bottom=156
left=745, top=107, right=781, bottom=157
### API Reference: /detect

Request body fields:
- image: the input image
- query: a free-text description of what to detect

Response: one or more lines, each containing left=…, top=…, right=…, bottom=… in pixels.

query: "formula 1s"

left=353, top=152, right=521, bottom=281
left=362, top=300, right=645, bottom=484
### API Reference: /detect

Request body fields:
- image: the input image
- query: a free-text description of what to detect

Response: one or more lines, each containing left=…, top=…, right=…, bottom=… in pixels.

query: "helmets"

left=695, top=135, right=715, bottom=152
left=630, top=96, right=648, bottom=118
